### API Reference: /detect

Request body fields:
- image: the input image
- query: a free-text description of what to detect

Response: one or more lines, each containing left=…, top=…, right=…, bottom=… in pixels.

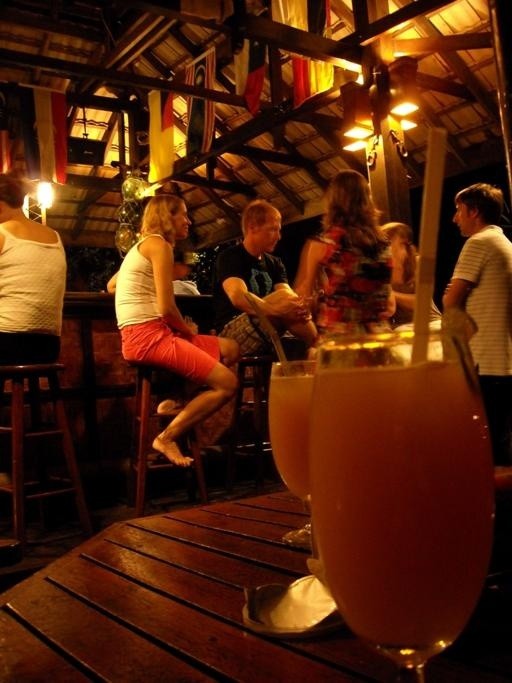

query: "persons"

left=256, top=168, right=397, bottom=551
left=375, top=218, right=443, bottom=323
left=206, top=195, right=321, bottom=354
left=107, top=194, right=242, bottom=471
left=443, top=182, right=511, bottom=594
left=0, top=173, right=66, bottom=485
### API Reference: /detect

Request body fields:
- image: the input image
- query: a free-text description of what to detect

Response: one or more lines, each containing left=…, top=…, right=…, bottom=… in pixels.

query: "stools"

left=224, top=354, right=282, bottom=494
left=122, top=357, right=210, bottom=517
left=1, top=359, right=96, bottom=545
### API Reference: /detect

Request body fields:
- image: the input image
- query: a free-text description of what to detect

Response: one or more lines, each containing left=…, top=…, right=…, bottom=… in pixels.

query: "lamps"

left=380, top=53, right=423, bottom=118
left=337, top=76, right=375, bottom=141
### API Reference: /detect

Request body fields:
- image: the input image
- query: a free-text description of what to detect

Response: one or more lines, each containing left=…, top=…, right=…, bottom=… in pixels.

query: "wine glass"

left=311, top=332, right=497, bottom=683
left=267, top=357, right=312, bottom=550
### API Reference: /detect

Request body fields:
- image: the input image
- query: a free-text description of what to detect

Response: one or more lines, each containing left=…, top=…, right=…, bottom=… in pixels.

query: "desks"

left=1, top=478, right=512, bottom=681
